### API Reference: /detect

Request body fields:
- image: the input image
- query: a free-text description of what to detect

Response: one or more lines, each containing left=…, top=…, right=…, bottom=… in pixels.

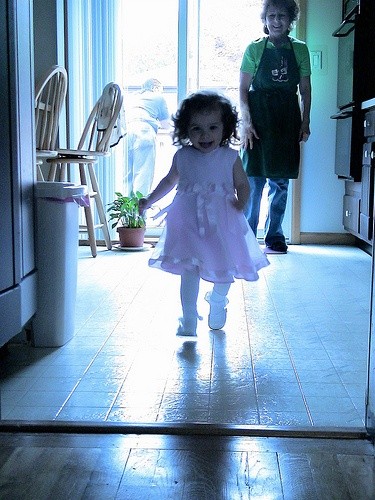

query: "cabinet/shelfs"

left=0, top=0, right=38, bottom=350
left=332, top=0, right=375, bottom=256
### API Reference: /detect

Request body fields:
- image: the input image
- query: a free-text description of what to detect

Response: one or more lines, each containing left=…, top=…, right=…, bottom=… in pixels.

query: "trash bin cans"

left=35, top=181, right=88, bottom=347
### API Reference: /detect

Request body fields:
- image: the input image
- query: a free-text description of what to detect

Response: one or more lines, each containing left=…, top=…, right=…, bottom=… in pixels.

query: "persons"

left=138, top=91, right=272, bottom=337
left=237, top=0, right=311, bottom=252
left=123, top=78, right=170, bottom=198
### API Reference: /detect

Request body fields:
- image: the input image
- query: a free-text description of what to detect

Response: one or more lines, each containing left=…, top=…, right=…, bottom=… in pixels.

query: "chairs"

left=35, top=64, right=69, bottom=182
left=46, top=80, right=123, bottom=257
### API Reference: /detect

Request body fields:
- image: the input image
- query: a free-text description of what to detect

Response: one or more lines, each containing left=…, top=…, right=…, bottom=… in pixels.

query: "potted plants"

left=106, top=191, right=154, bottom=247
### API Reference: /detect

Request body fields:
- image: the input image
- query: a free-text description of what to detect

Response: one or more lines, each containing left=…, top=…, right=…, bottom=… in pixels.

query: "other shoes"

left=271, top=242, right=288, bottom=253
left=208, top=301, right=226, bottom=331
left=176, top=323, right=199, bottom=337
